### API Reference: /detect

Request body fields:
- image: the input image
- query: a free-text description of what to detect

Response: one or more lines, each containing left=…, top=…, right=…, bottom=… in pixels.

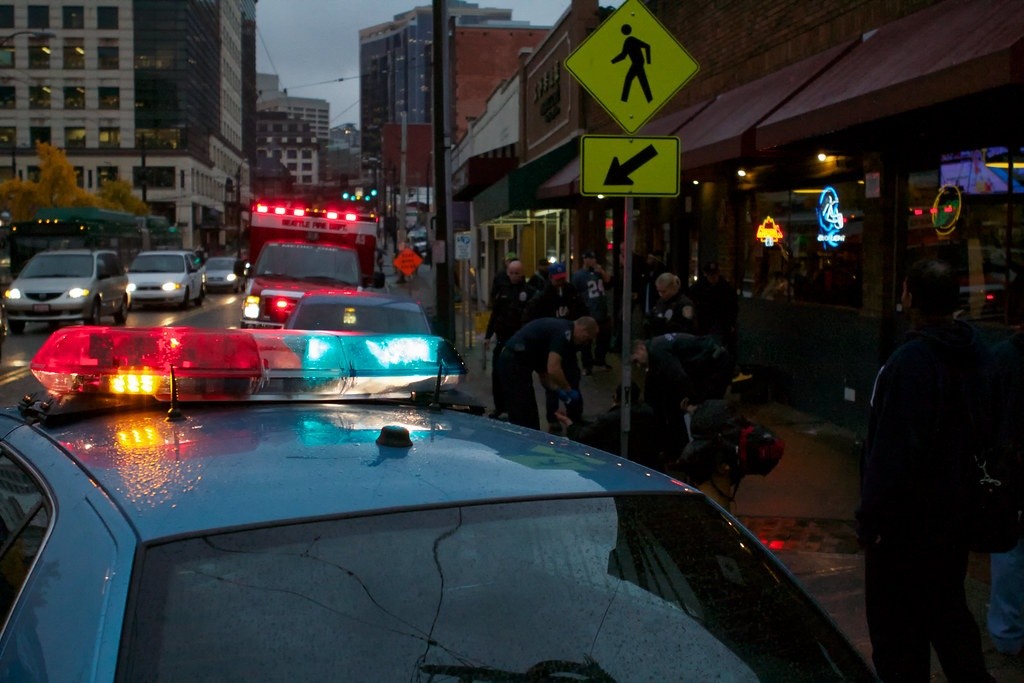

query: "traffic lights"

left=339, top=181, right=352, bottom=201
left=364, top=189, right=371, bottom=202
left=10, top=205, right=185, bottom=283
left=370, top=181, right=378, bottom=197
left=349, top=188, right=357, bottom=202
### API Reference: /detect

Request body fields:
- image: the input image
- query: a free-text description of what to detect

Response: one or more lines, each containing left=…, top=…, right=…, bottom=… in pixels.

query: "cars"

left=281, top=292, right=438, bottom=338
left=2, top=249, right=133, bottom=333
left=0, top=325, right=892, bottom=683
left=205, top=257, right=246, bottom=292
left=123, top=249, right=207, bottom=311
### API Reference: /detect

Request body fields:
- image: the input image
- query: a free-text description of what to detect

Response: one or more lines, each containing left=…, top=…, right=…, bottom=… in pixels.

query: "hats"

left=547, top=263, right=567, bottom=286
left=538, top=259, right=550, bottom=266
left=505, top=252, right=518, bottom=261
left=703, top=261, right=720, bottom=275
left=581, top=250, right=596, bottom=259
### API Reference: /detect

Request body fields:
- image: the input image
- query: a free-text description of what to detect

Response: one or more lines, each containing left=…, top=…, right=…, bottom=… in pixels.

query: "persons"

left=857, top=267, right=1024, bottom=681
left=483, top=252, right=799, bottom=515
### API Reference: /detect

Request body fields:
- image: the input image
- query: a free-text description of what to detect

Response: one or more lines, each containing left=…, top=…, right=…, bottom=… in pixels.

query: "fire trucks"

left=241, top=204, right=386, bottom=330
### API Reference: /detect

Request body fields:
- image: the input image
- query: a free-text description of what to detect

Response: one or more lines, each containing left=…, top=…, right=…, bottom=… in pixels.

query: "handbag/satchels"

left=971, top=474, right=1020, bottom=554
left=708, top=413, right=786, bottom=477
